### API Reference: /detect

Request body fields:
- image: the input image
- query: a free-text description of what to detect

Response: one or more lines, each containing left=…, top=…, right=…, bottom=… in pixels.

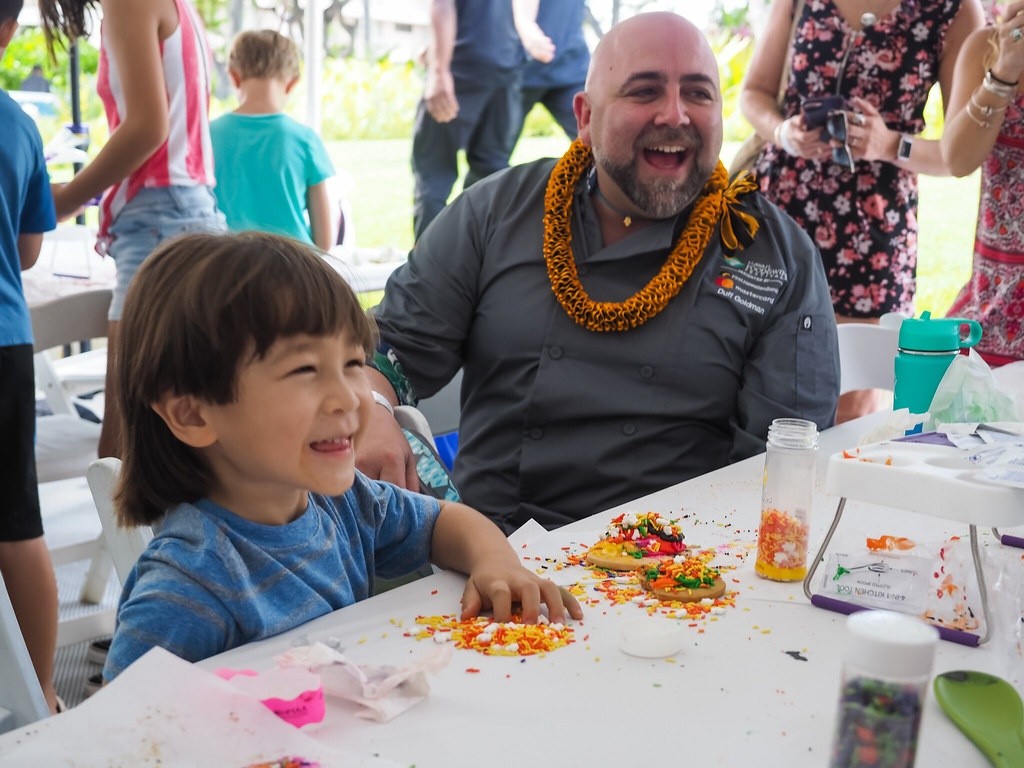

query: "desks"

left=1, top=408, right=1024, bottom=768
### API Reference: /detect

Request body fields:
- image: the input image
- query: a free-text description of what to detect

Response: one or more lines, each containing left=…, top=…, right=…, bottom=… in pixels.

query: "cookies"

left=640, top=578, right=726, bottom=602
left=587, top=538, right=693, bottom=571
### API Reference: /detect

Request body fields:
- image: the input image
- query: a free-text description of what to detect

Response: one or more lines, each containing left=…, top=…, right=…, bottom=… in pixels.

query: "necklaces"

left=861, top=0, right=889, bottom=27
left=597, top=189, right=659, bottom=226
left=541, top=135, right=729, bottom=333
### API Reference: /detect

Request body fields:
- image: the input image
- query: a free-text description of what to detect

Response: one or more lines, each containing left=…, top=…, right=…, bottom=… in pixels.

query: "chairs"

left=27, top=288, right=443, bottom=649
left=833, top=322, right=901, bottom=396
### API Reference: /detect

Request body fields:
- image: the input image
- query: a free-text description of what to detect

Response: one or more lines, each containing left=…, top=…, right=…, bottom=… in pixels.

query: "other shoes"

left=83, top=671, right=104, bottom=691
left=86, top=638, right=112, bottom=664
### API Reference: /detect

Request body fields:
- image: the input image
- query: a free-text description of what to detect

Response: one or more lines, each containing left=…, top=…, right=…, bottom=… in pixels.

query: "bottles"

left=894, top=311, right=983, bottom=414
left=829, top=610, right=938, bottom=768
left=756, top=418, right=820, bottom=582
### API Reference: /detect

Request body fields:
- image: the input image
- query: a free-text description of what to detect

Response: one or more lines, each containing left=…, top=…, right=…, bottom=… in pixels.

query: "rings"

left=851, top=138, right=857, bottom=146
left=1010, top=28, right=1022, bottom=41
left=817, top=147, right=822, bottom=154
left=853, top=113, right=864, bottom=125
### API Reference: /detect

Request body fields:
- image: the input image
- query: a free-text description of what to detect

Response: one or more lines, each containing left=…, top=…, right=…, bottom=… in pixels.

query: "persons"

left=209, top=31, right=337, bottom=258
left=409, top=0, right=603, bottom=247
left=939, top=0, right=1024, bottom=374
left=354, top=12, right=840, bottom=539
left=0, top=0, right=66, bottom=719
left=738, top=0, right=988, bottom=426
left=36, top=0, right=228, bottom=699
left=99, top=231, right=584, bottom=691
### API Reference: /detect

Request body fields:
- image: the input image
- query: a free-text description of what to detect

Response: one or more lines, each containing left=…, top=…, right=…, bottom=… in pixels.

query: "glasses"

left=826, top=111, right=854, bottom=173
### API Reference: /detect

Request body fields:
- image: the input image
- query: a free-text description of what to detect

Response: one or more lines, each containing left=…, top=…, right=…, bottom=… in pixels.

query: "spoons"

left=933, top=670, right=1024, bottom=768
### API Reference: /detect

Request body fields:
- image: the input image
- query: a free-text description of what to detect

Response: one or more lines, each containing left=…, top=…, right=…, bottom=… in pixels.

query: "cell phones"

left=803, top=96, right=847, bottom=142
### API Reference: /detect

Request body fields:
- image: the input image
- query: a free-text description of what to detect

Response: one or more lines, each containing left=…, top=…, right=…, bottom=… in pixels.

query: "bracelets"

left=371, top=392, right=394, bottom=414
left=983, top=69, right=1020, bottom=98
left=966, top=88, right=1007, bottom=128
left=774, top=123, right=783, bottom=145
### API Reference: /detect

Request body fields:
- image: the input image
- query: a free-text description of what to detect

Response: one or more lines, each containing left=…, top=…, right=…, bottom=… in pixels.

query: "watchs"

left=891, top=132, right=914, bottom=165
